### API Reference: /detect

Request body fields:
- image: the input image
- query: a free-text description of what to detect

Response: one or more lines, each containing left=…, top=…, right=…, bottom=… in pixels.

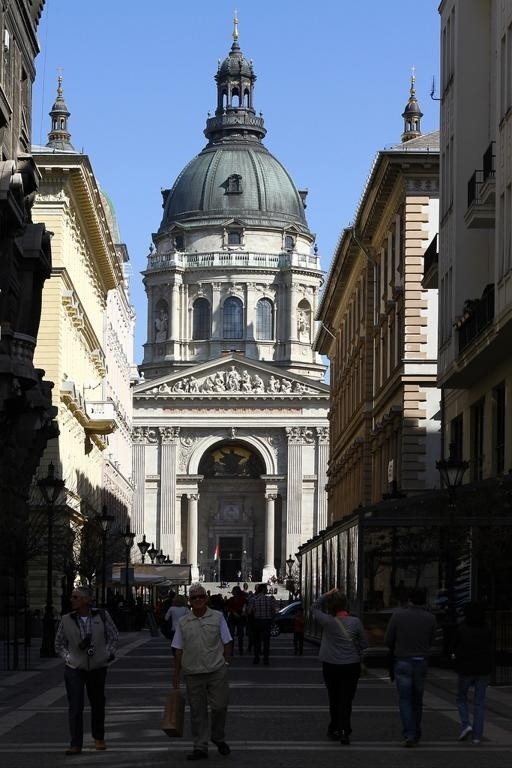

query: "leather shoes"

left=65, top=747, right=82, bottom=755
left=211, top=738, right=230, bottom=756
left=95, top=740, right=106, bottom=750
left=187, top=750, right=209, bottom=761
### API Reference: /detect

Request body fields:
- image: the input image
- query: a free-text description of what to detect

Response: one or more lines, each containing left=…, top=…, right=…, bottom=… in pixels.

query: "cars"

left=270, top=601, right=302, bottom=637
left=359, top=607, right=406, bottom=654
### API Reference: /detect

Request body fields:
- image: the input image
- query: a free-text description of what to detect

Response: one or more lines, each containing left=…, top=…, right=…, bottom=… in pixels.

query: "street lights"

left=285, top=553, right=295, bottom=579
left=435, top=453, right=470, bottom=671
left=121, top=523, right=173, bottom=604
left=294, top=545, right=302, bottom=592
left=94, top=502, right=115, bottom=609
left=37, top=459, right=65, bottom=662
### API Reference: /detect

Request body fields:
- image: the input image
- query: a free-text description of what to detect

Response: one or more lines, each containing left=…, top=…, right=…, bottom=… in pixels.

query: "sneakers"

left=473, top=740, right=481, bottom=744
left=457, top=726, right=473, bottom=742
left=327, top=731, right=342, bottom=741
left=341, top=737, right=350, bottom=745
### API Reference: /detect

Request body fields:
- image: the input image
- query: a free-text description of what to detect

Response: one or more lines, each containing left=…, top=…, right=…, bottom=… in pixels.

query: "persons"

left=450, top=601, right=495, bottom=747
left=54, top=585, right=121, bottom=754
left=289, top=607, right=306, bottom=655
left=156, top=580, right=275, bottom=666
left=169, top=583, right=239, bottom=761
left=307, top=587, right=368, bottom=747
left=382, top=586, right=434, bottom=748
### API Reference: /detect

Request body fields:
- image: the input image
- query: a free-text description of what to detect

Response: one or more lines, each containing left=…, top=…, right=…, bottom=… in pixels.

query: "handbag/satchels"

left=160, top=670, right=185, bottom=738
left=359, top=664, right=368, bottom=677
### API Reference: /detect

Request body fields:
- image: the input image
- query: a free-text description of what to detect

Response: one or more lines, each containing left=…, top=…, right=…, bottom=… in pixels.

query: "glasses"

left=189, top=593, right=208, bottom=600
